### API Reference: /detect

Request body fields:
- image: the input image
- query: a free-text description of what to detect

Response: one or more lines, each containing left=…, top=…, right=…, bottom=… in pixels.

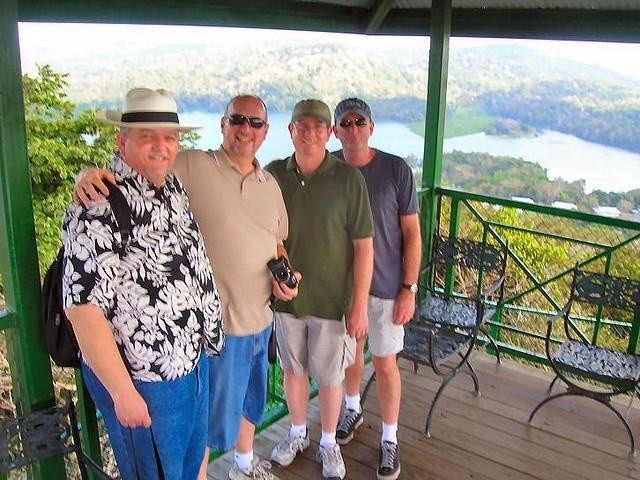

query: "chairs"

left=526, top=261, right=639, bottom=454
left=359, top=228, right=509, bottom=439
left=0, top=387, right=110, bottom=480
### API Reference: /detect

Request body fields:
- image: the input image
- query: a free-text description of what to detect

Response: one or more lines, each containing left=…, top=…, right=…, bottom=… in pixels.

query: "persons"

left=74, top=95, right=289, bottom=479
left=264, top=99, right=375, bottom=479
left=62, top=86, right=225, bottom=479
left=330, top=97, right=426, bottom=479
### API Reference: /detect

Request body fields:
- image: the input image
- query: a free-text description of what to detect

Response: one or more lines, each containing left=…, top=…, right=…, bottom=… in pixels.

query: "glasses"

left=291, top=121, right=330, bottom=134
left=338, top=116, right=370, bottom=128
left=221, top=112, right=268, bottom=128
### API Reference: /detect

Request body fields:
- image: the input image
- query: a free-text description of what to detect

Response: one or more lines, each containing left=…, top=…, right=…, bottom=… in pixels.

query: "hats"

left=333, top=97, right=372, bottom=126
left=291, top=99, right=332, bottom=125
left=103, top=87, right=204, bottom=128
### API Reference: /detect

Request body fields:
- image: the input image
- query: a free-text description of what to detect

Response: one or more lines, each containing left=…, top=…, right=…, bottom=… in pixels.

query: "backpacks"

left=42, top=176, right=131, bottom=366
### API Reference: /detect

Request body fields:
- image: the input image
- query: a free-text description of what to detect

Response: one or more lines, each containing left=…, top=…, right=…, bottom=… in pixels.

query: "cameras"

left=268, top=256, right=297, bottom=290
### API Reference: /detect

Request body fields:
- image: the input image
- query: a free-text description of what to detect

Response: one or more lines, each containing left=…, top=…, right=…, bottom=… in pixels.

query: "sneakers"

left=267, top=425, right=309, bottom=467
left=375, top=433, right=400, bottom=478
left=227, top=457, right=283, bottom=480
left=315, top=441, right=346, bottom=479
left=334, top=399, right=365, bottom=446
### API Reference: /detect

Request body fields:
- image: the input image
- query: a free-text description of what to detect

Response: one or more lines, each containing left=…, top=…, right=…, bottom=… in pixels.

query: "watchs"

left=402, top=283, right=418, bottom=293
